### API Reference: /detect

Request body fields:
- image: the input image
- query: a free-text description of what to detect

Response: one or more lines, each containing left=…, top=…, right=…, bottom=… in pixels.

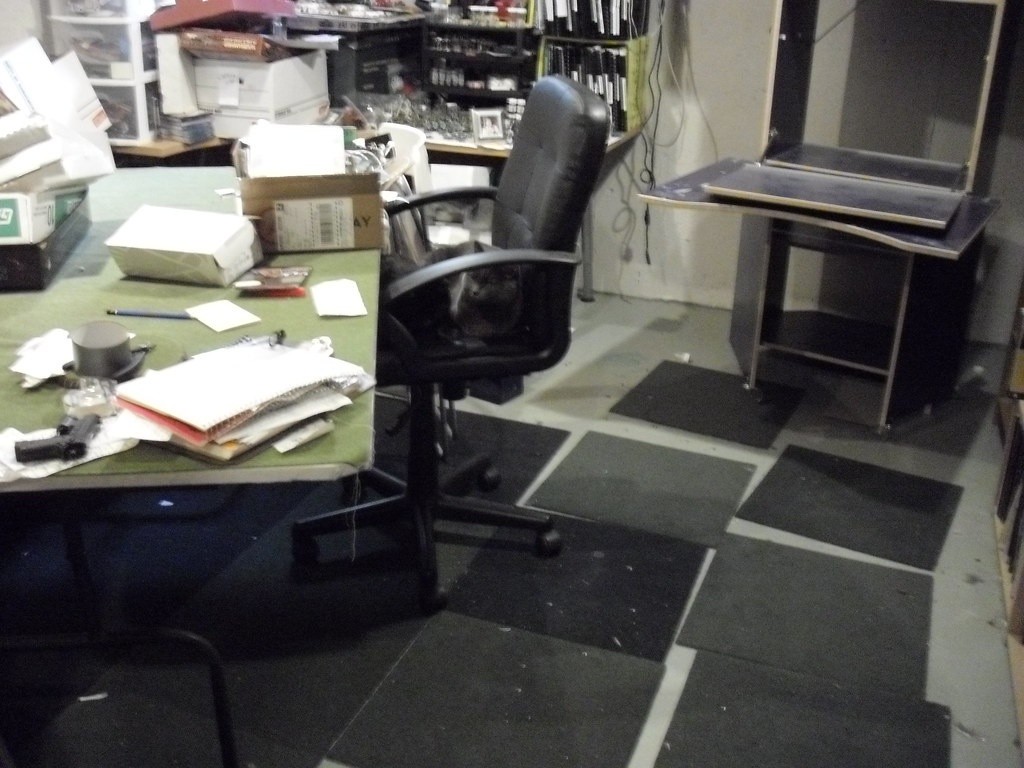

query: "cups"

left=72, top=320, right=133, bottom=378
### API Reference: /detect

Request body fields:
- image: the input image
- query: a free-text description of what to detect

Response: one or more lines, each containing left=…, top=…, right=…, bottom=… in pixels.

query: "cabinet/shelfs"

left=990, top=273, right=1024, bottom=766
left=636, top=0, right=1004, bottom=431
left=47, top=0, right=157, bottom=146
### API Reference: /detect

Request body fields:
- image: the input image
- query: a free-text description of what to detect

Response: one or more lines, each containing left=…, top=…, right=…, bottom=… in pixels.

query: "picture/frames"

left=469, top=107, right=508, bottom=145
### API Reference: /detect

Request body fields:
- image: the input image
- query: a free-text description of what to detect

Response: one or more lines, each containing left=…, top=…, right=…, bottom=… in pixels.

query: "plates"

left=63, top=350, right=146, bottom=380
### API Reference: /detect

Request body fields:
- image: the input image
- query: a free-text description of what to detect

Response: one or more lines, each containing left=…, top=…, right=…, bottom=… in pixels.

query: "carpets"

left=0, top=356, right=964, bottom=768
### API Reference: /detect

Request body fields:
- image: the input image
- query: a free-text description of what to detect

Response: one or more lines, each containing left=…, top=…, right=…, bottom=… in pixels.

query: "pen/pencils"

left=106, top=308, right=195, bottom=319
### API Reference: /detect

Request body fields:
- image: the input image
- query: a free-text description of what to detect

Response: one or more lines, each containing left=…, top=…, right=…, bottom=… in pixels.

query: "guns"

left=12, top=412, right=102, bottom=464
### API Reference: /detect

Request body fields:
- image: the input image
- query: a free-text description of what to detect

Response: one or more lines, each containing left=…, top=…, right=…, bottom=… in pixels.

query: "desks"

left=0, top=159, right=390, bottom=768
left=114, top=125, right=645, bottom=304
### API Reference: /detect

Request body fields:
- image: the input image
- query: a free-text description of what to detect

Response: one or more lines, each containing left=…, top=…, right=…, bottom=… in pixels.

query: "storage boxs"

left=0, top=184, right=93, bottom=293
left=234, top=172, right=383, bottom=254
left=231, top=120, right=345, bottom=178
left=151, top=0, right=330, bottom=143
left=109, top=203, right=263, bottom=288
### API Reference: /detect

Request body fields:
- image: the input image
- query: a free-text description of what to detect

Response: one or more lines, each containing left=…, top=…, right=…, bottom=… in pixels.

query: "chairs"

left=284, top=74, right=616, bottom=613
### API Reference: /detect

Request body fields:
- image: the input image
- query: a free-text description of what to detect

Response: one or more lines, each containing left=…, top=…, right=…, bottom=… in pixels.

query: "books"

left=115, top=335, right=376, bottom=467
